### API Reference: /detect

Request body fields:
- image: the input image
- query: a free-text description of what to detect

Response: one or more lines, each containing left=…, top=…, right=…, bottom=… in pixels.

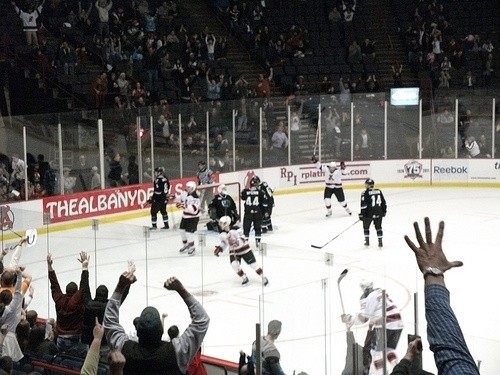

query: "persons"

left=311, top=154, right=353, bottom=217
left=204, top=184, right=239, bottom=233
left=196, top=161, right=216, bottom=214
left=340, top=278, right=403, bottom=375
left=175, top=181, right=203, bottom=256
left=403, top=216, right=480, bottom=375
left=146, top=167, right=171, bottom=231
left=390, top=337, right=435, bottom=375
left=0, top=0, right=500, bottom=205
left=102, top=270, right=210, bottom=375
left=357, top=177, right=388, bottom=249
left=0, top=236, right=208, bottom=375
left=11, top=0, right=46, bottom=48
left=240, top=179, right=268, bottom=249
left=214, top=215, right=270, bottom=287
left=252, top=320, right=285, bottom=375
left=252, top=176, right=274, bottom=236
left=341, top=320, right=377, bottom=375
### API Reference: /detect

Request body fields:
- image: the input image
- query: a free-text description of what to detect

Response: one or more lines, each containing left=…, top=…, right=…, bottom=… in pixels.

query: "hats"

left=137, top=307, right=162, bottom=336
left=198, top=160, right=205, bottom=164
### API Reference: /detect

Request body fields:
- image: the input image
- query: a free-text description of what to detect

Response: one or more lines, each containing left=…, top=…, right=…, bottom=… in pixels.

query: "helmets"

left=359, top=277, right=373, bottom=290
left=364, top=178, right=374, bottom=185
left=155, top=167, right=163, bottom=175
left=219, top=216, right=231, bottom=224
left=186, top=181, right=196, bottom=192
left=250, top=176, right=260, bottom=186
left=328, top=162, right=336, bottom=167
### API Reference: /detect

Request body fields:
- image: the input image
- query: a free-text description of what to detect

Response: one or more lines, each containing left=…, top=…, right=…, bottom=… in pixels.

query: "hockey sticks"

left=311, top=220, right=360, bottom=250
left=169, top=200, right=178, bottom=230
left=337, top=269, right=350, bottom=333
left=313, top=107, right=326, bottom=162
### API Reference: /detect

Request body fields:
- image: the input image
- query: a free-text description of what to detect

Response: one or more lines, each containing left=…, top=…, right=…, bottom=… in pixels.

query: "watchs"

left=424, top=266, right=444, bottom=278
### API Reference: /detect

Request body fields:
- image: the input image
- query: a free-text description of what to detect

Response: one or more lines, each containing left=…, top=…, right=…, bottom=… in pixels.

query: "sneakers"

left=326, top=211, right=331, bottom=216
left=179, top=246, right=187, bottom=254
left=378, top=238, right=383, bottom=247
left=242, top=277, right=248, bottom=284
left=364, top=238, right=369, bottom=245
left=263, top=277, right=268, bottom=286
left=346, top=209, right=351, bottom=215
left=188, top=247, right=194, bottom=255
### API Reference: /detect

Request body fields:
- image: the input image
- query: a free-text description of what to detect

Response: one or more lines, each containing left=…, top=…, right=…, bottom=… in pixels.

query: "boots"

left=150, top=223, right=157, bottom=230
left=161, top=222, right=169, bottom=230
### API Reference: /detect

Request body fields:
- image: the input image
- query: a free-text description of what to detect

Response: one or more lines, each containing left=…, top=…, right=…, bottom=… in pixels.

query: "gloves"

left=312, top=155, right=317, bottom=163
left=214, top=246, right=222, bottom=256
left=381, top=212, right=385, bottom=217
left=359, top=213, right=363, bottom=220
left=340, top=161, right=345, bottom=169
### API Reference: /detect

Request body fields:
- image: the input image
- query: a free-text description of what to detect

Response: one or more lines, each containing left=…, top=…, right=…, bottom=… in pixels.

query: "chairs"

left=0, top=0, right=500, bottom=115
